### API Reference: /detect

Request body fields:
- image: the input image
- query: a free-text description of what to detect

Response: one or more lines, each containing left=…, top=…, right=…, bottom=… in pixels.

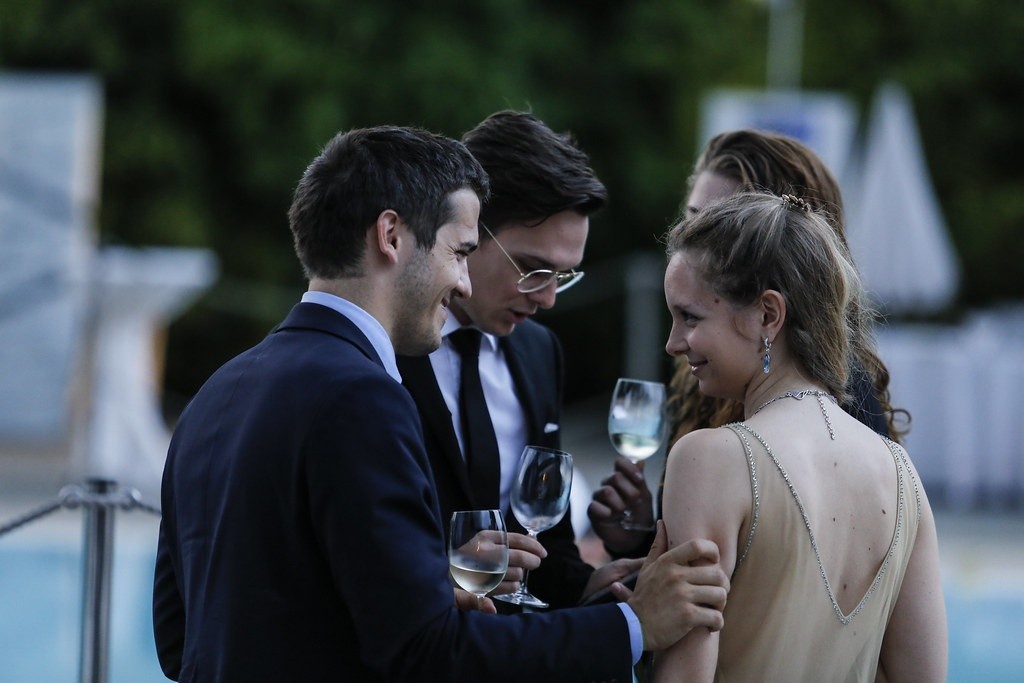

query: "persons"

left=150, top=110, right=732, bottom=683
left=587, top=129, right=950, bottom=683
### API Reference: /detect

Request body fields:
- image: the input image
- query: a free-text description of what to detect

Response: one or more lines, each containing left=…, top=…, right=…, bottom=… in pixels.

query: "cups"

left=448, top=507, right=510, bottom=612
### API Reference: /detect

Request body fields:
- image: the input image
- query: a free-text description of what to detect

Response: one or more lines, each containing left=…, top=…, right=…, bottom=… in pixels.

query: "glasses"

left=479, top=220, right=584, bottom=293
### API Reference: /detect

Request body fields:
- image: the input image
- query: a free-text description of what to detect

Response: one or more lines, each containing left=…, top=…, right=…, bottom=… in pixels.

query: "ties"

left=447, top=328, right=501, bottom=508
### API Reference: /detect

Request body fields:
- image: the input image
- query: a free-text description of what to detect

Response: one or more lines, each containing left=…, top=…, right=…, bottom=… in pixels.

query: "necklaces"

left=754, top=389, right=836, bottom=440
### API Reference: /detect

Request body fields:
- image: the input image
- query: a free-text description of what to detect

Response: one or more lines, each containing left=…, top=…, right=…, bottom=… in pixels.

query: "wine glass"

left=596, top=377, right=669, bottom=533
left=491, top=445, right=575, bottom=609
